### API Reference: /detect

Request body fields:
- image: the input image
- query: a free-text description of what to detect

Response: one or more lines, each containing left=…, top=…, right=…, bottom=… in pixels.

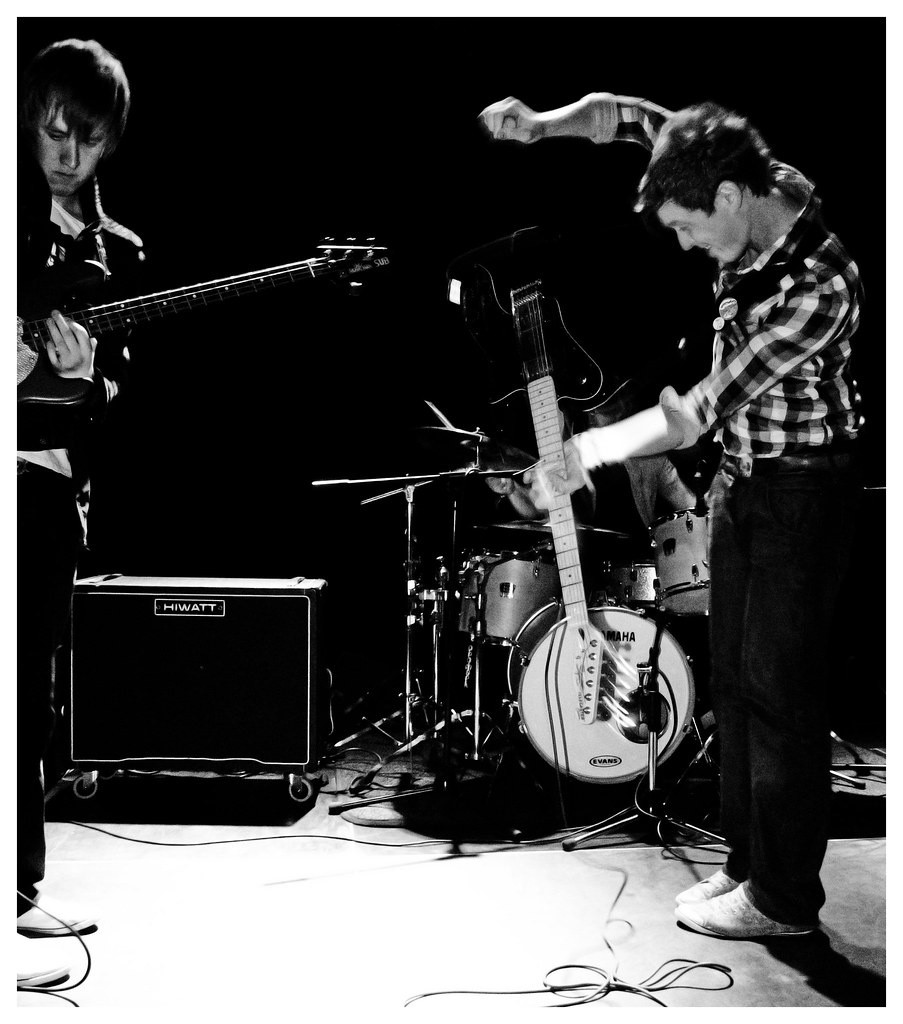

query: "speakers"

left=67, top=572, right=330, bottom=774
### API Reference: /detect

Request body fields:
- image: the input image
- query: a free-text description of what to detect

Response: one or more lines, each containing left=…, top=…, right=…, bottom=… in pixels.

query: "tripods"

left=563, top=605, right=729, bottom=858
left=312, top=437, right=512, bottom=841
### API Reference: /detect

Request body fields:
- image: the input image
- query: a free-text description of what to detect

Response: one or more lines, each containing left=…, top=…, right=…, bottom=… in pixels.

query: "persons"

left=18, top=40, right=146, bottom=987
left=480, top=94, right=872, bottom=940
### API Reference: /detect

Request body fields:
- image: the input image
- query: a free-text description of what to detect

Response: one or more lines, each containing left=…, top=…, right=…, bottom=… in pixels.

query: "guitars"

left=506, top=279, right=610, bottom=725
left=16, top=235, right=389, bottom=408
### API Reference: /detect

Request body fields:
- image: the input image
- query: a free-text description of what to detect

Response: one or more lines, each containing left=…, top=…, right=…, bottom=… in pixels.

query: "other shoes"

left=17, top=894, right=99, bottom=935
left=674, top=882, right=814, bottom=939
left=14, top=931, right=71, bottom=987
left=674, top=868, right=744, bottom=907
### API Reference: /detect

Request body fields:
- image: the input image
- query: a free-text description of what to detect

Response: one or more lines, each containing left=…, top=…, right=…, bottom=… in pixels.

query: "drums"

left=647, top=506, right=710, bottom=617
left=458, top=549, right=559, bottom=650
left=507, top=601, right=695, bottom=787
left=616, top=561, right=657, bottom=605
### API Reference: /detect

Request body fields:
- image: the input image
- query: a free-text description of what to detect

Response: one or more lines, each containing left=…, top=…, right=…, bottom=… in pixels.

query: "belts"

left=727, top=455, right=809, bottom=478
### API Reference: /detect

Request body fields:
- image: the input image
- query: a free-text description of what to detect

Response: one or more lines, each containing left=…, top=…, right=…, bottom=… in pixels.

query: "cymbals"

left=421, top=422, right=537, bottom=461
left=493, top=516, right=628, bottom=541
